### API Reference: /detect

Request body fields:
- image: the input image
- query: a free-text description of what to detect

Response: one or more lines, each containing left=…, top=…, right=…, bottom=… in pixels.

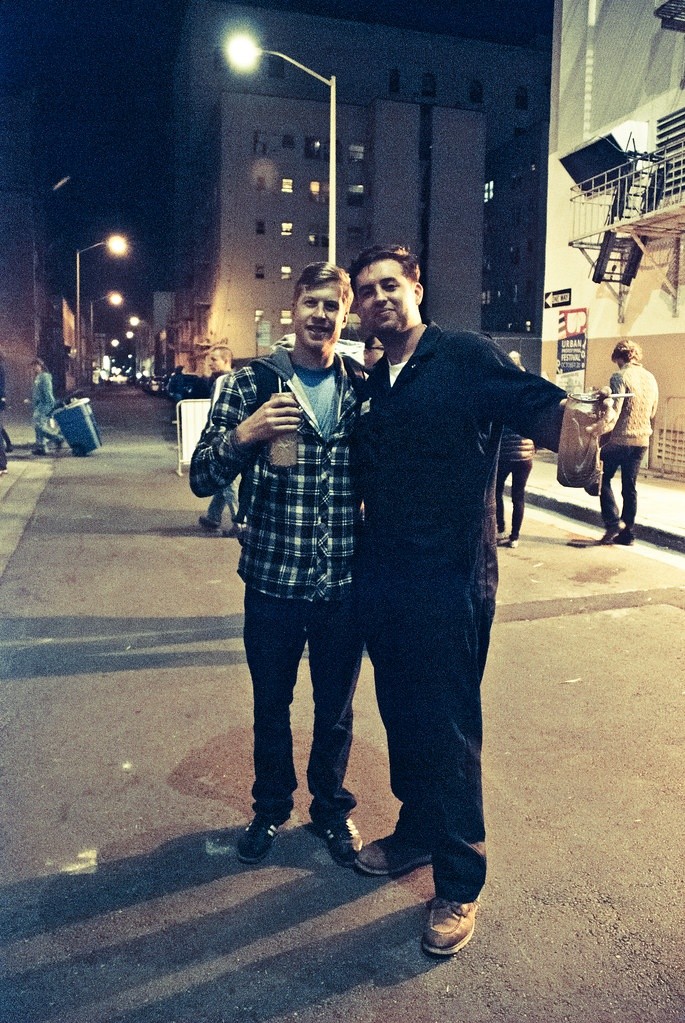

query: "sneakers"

left=235, top=815, right=281, bottom=865
left=601, top=527, right=620, bottom=544
left=614, top=530, right=635, bottom=544
left=324, top=818, right=363, bottom=867
left=354, top=832, right=432, bottom=876
left=496, top=534, right=508, bottom=546
left=509, top=540, right=519, bottom=549
left=421, top=896, right=479, bottom=955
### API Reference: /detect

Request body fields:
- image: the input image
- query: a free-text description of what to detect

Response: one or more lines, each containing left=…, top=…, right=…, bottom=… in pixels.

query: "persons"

left=31, top=358, right=64, bottom=455
left=363, top=333, right=386, bottom=366
left=199, top=346, right=243, bottom=538
left=189, top=262, right=368, bottom=867
left=169, top=365, right=191, bottom=424
left=0, top=351, right=13, bottom=474
left=496, top=352, right=534, bottom=548
left=599, top=339, right=659, bottom=545
left=345, top=244, right=617, bottom=955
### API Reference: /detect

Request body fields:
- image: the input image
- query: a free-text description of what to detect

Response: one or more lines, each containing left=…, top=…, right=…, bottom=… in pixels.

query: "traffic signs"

left=545, top=289, right=572, bottom=309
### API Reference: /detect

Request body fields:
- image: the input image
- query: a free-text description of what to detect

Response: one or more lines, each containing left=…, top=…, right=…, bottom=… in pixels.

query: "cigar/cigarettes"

left=596, top=394, right=635, bottom=398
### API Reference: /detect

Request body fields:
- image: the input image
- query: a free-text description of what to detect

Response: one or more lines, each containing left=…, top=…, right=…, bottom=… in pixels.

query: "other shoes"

left=32, top=449, right=46, bottom=455
left=56, top=438, right=64, bottom=449
left=0, top=468, right=8, bottom=475
left=5, top=446, right=13, bottom=452
left=199, top=515, right=220, bottom=529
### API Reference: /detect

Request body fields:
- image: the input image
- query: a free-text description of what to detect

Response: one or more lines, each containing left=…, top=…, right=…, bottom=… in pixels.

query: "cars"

left=128, top=373, right=212, bottom=401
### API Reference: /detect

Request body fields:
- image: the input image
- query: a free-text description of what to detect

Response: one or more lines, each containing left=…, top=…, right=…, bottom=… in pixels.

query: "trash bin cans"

left=46, top=389, right=103, bottom=456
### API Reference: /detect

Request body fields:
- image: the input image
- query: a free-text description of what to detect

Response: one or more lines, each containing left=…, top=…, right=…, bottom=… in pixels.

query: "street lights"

left=99, top=338, right=119, bottom=369
left=76, top=235, right=128, bottom=392
left=222, top=33, right=336, bottom=266
left=126, top=317, right=153, bottom=374
left=90, top=292, right=123, bottom=387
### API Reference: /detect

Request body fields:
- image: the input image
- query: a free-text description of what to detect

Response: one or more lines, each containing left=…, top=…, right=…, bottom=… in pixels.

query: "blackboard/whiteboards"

left=180, top=402, right=212, bottom=465
